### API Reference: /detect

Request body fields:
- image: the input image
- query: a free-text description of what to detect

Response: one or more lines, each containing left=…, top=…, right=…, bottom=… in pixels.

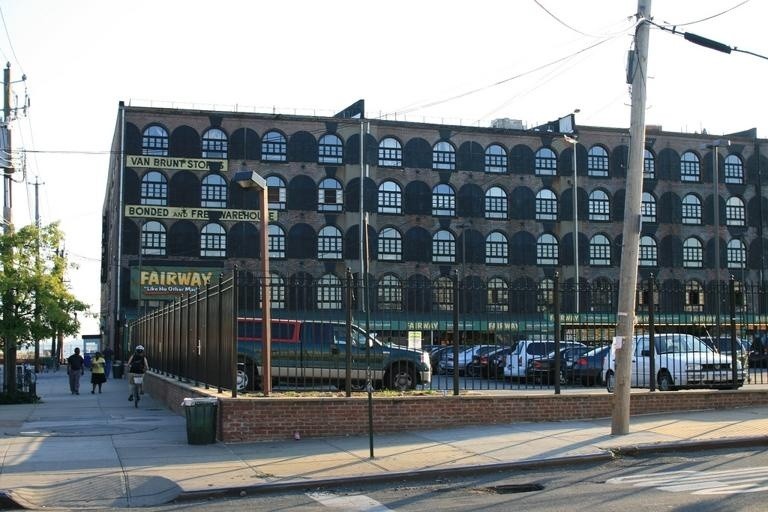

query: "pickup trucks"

left=236, top=321, right=433, bottom=394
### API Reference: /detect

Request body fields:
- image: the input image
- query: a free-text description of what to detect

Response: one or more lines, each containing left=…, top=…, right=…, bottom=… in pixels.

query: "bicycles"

left=123, top=364, right=149, bottom=409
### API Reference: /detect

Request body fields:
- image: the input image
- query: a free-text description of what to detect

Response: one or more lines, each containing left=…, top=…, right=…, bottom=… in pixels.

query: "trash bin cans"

left=181, top=397, right=220, bottom=445
left=112, top=360, right=123, bottom=379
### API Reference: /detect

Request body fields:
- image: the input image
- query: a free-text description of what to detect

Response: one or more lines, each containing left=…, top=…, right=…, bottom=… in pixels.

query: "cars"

left=238, top=317, right=301, bottom=342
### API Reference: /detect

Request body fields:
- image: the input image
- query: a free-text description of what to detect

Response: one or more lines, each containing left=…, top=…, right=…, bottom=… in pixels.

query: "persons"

left=103, top=345, right=113, bottom=378
left=67, top=348, right=85, bottom=394
left=91, top=352, right=106, bottom=394
left=127, top=345, right=149, bottom=400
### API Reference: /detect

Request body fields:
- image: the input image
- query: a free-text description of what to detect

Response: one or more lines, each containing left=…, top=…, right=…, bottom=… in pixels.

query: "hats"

left=135, top=345, right=144, bottom=350
